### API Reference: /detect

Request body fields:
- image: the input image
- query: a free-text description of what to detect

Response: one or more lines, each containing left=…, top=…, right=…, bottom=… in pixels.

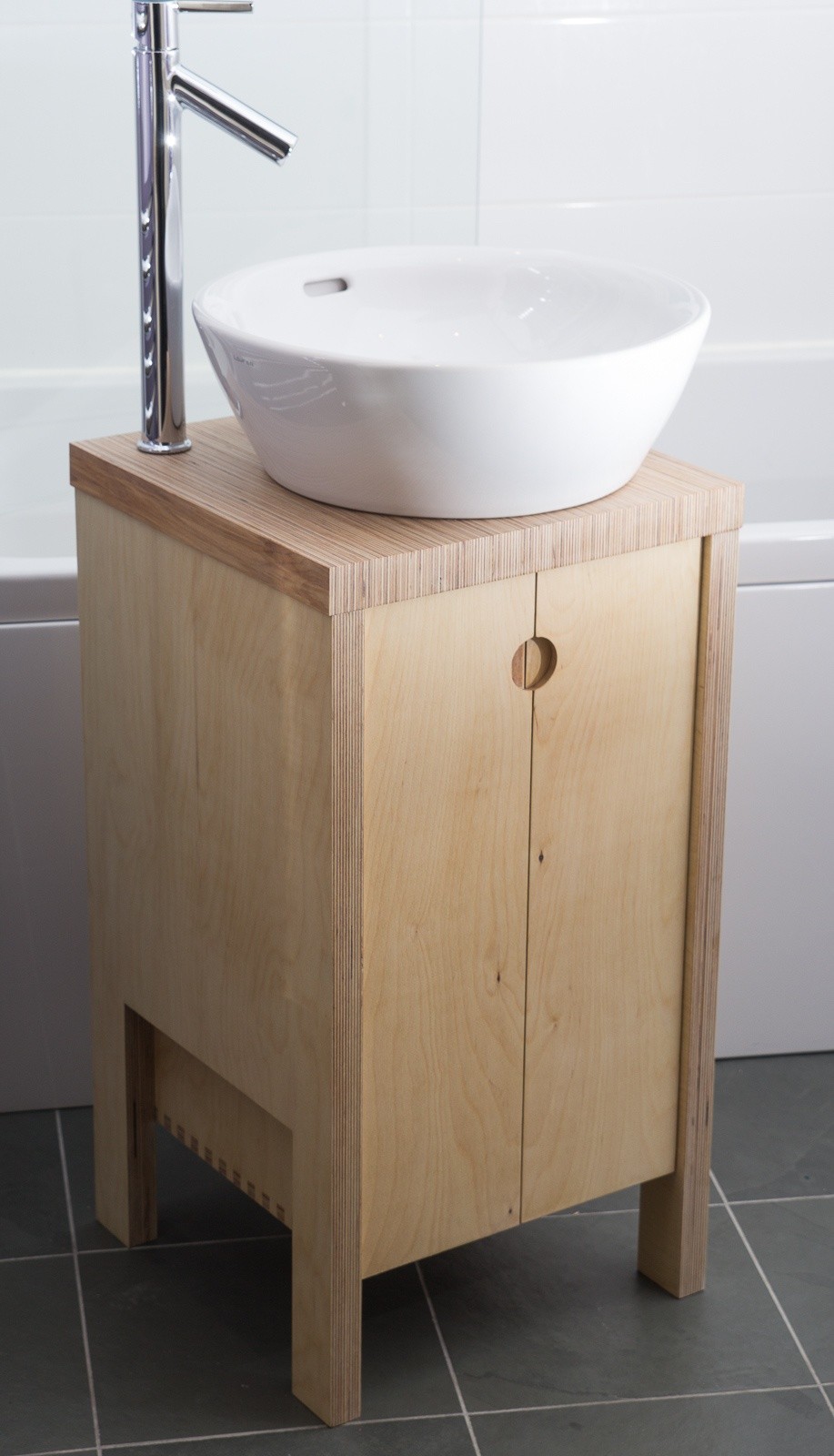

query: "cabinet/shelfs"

left=70, top=416, right=745, bottom=1425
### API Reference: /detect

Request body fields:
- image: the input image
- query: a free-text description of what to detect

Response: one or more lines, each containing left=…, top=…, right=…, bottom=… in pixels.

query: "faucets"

left=124, top=0, right=299, bottom=460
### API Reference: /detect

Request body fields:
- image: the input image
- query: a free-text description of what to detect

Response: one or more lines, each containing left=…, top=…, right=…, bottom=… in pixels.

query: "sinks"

left=188, top=241, right=712, bottom=521
